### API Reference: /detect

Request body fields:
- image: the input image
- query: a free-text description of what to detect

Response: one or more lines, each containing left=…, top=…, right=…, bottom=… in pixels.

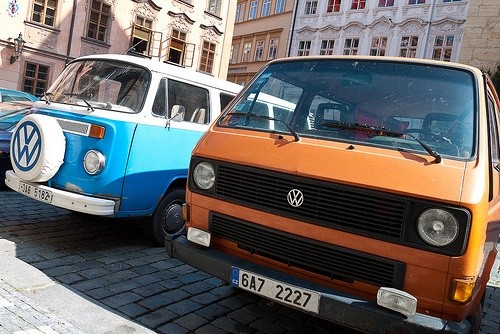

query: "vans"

left=166, top=56, right=500, bottom=331
left=4, top=21, right=317, bottom=247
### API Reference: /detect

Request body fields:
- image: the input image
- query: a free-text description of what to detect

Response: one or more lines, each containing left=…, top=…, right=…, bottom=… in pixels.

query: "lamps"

left=8, top=31, right=25, bottom=64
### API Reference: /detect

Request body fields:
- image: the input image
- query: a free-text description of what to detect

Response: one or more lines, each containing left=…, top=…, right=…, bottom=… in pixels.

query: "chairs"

left=416, top=113, right=464, bottom=155
left=304, top=103, right=352, bottom=141
